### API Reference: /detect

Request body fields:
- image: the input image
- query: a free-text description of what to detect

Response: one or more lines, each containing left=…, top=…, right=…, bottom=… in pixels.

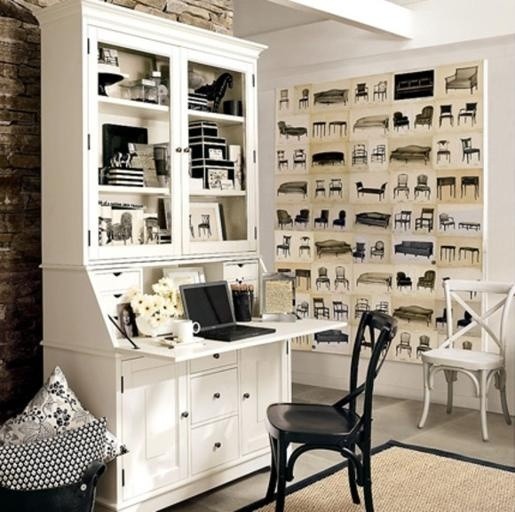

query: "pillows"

left=0, top=365, right=128, bottom=492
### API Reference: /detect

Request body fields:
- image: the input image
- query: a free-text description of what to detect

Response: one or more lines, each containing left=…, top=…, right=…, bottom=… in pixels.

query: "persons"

left=120, top=306, right=138, bottom=337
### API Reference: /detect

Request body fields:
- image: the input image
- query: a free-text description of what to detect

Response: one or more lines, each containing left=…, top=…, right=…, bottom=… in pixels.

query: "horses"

left=188, top=72, right=234, bottom=113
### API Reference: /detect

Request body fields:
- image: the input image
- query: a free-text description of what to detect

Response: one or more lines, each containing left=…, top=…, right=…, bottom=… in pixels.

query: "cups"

left=170, top=320, right=200, bottom=344
left=223, top=100, right=243, bottom=116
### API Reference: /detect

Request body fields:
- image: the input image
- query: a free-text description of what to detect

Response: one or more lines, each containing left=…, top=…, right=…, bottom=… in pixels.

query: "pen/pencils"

left=230, top=284, right=257, bottom=291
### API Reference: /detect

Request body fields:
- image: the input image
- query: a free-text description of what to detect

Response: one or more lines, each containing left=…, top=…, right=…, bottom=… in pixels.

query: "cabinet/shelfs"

left=34, top=1, right=349, bottom=512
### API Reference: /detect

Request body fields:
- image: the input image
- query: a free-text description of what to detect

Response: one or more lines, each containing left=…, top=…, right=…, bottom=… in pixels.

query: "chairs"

left=261, top=309, right=399, bottom=512
left=413, top=276, right=514, bottom=442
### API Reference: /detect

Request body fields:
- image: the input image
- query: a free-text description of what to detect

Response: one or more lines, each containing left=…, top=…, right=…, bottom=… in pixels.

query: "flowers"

left=125, top=278, right=183, bottom=326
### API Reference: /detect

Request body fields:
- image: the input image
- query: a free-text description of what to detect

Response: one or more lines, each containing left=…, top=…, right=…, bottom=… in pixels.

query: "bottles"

left=130, top=71, right=162, bottom=104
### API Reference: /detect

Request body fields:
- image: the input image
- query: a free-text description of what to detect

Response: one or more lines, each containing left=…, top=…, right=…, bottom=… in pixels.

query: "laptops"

left=180, top=279, right=276, bottom=344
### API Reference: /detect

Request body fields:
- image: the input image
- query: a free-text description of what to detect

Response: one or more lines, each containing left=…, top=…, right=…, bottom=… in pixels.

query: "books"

left=103, top=124, right=170, bottom=187
